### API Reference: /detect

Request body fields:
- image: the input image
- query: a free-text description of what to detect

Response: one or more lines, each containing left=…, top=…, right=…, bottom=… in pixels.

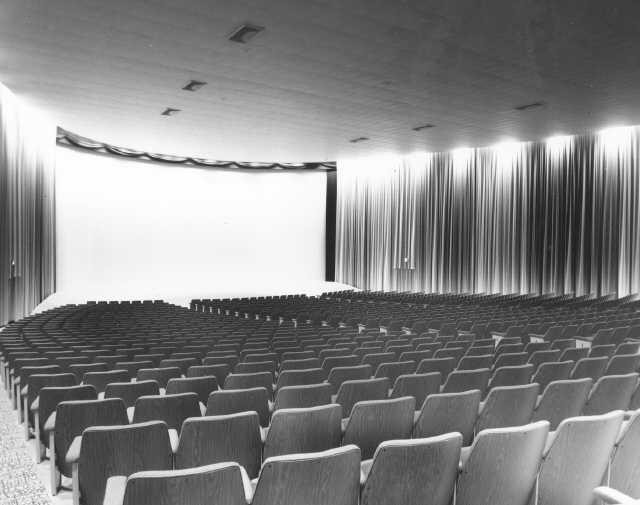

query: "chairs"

left=2, top=285, right=639, bottom=504
